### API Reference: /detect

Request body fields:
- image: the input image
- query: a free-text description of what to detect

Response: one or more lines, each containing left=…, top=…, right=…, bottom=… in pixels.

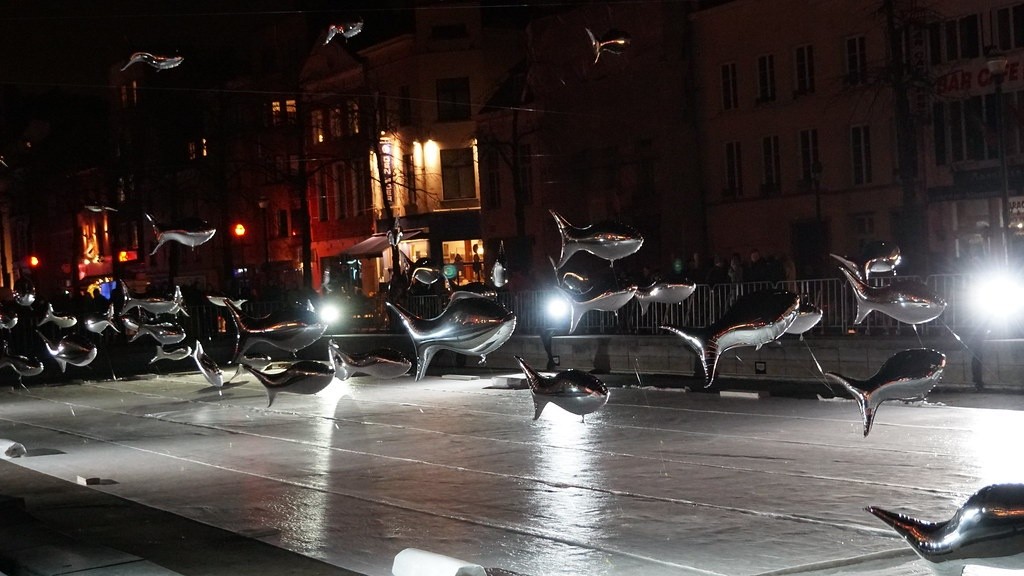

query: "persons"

left=13, top=267, right=35, bottom=353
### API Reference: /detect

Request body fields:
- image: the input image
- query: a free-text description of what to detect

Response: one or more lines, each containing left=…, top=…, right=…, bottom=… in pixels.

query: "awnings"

left=339, top=229, right=423, bottom=260
